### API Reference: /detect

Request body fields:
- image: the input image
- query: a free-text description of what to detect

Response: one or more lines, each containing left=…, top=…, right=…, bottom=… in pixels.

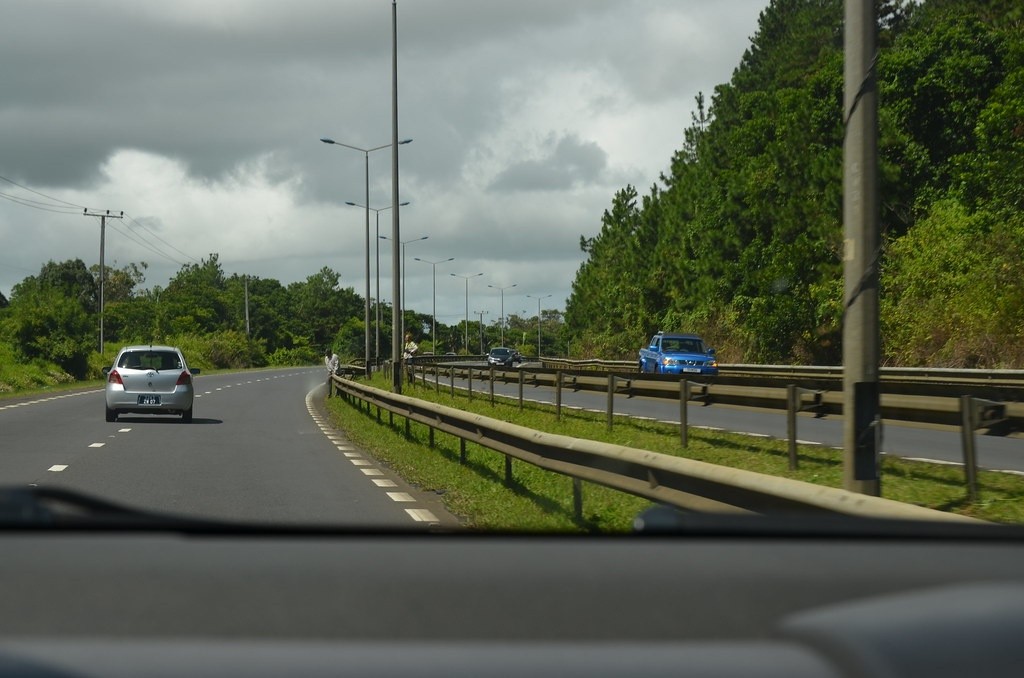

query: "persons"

left=325, top=348, right=340, bottom=399
left=404, top=334, right=418, bottom=384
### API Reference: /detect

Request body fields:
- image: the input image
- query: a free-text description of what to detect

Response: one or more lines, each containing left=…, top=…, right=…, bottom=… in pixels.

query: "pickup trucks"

left=639, top=335, right=717, bottom=380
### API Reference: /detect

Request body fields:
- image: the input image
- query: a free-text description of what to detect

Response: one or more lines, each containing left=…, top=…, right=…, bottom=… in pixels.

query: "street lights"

left=488, top=283, right=518, bottom=346
left=450, top=272, right=483, bottom=353
left=526, top=294, right=551, bottom=358
left=379, top=234, right=429, bottom=359
left=412, top=257, right=454, bottom=358
left=345, top=201, right=409, bottom=371
left=320, top=136, right=412, bottom=372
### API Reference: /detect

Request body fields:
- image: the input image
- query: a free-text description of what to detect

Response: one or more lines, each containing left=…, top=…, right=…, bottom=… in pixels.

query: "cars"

left=101, top=344, right=200, bottom=422
left=486, top=348, right=512, bottom=368
left=511, top=350, right=523, bottom=364
